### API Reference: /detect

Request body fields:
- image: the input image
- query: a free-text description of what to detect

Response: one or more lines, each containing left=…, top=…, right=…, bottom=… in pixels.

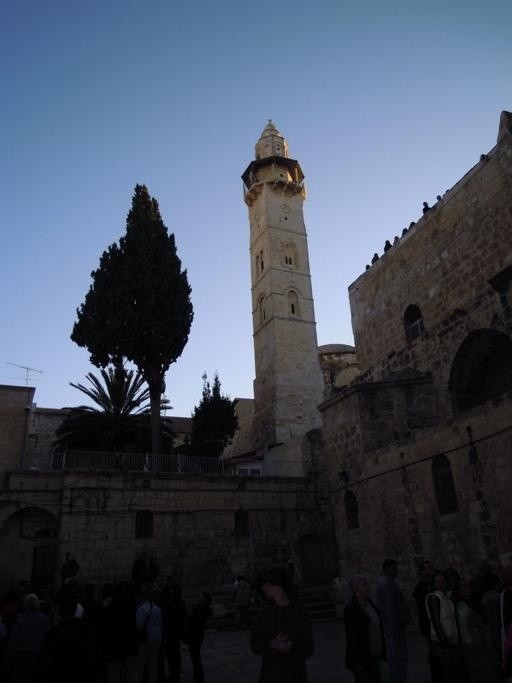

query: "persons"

left=343, top=559, right=414, bottom=683
left=366, top=263, right=370, bottom=270
left=230, top=570, right=274, bottom=628
left=402, top=228, right=408, bottom=237
left=393, top=234, right=399, bottom=246
left=2, top=570, right=191, bottom=679
left=422, top=201, right=431, bottom=214
left=250, top=569, right=314, bottom=683
left=131, top=551, right=149, bottom=577
left=384, top=239, right=393, bottom=252
left=436, top=195, right=441, bottom=201
left=60, top=551, right=81, bottom=574
left=408, top=221, right=415, bottom=231
left=189, top=591, right=212, bottom=681
left=371, top=252, right=380, bottom=264
left=412, top=556, right=512, bottom=681
